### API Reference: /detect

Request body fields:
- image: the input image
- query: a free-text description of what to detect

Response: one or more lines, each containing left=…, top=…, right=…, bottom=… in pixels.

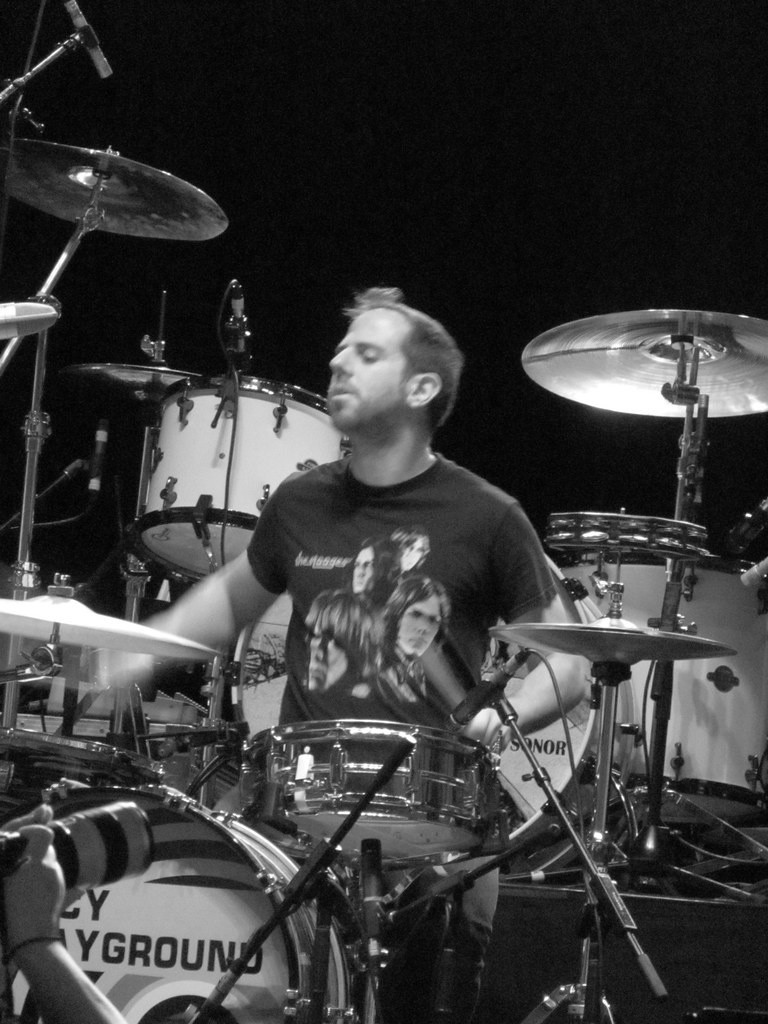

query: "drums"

left=488, top=546, right=768, bottom=825
left=129, top=372, right=350, bottom=582
left=0, top=784, right=353, bottom=1024
left=228, top=551, right=633, bottom=876
left=541, top=511, right=710, bottom=562
left=247, top=718, right=492, bottom=869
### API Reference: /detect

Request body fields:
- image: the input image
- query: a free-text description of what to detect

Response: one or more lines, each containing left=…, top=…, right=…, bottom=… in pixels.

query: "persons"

left=0, top=803, right=127, bottom=1024
left=92, top=289, right=591, bottom=1024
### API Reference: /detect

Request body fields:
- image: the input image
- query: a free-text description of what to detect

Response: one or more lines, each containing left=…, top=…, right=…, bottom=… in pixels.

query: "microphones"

left=740, top=556, right=768, bottom=587
left=450, top=648, right=531, bottom=727
left=231, top=281, right=245, bottom=353
left=88, top=417, right=110, bottom=503
left=63, top=0, right=113, bottom=78
left=723, top=498, right=768, bottom=554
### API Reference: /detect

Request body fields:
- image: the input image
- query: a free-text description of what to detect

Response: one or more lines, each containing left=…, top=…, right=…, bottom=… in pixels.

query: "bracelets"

left=10, top=937, right=59, bottom=954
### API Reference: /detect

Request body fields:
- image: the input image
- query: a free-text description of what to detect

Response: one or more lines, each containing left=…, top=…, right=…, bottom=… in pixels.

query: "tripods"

left=500, top=346, right=768, bottom=1024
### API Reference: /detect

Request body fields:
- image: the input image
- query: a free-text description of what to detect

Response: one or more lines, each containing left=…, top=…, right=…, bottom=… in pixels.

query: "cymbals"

left=57, top=360, right=204, bottom=390
left=687, top=530, right=708, bottom=554
left=486, top=616, right=738, bottom=663
left=580, top=518, right=610, bottom=542
left=656, top=526, right=686, bottom=549
left=520, top=308, right=768, bottom=419
left=618, top=519, right=649, bottom=544
left=545, top=518, right=576, bottom=542
left=0, top=596, right=223, bottom=662
left=0, top=135, right=230, bottom=242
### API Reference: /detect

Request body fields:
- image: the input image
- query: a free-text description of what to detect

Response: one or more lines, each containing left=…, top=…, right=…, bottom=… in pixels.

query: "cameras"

left=0, top=802, right=155, bottom=891
